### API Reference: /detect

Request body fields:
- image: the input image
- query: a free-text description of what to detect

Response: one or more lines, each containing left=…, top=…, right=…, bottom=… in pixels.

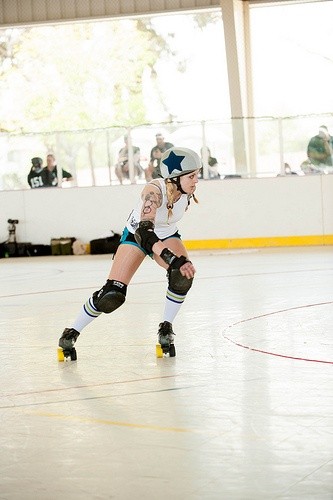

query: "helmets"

left=160, top=147, right=203, bottom=178
left=32, top=158, right=42, bottom=164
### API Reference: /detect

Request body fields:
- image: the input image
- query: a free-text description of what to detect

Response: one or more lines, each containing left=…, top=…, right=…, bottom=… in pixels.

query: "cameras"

left=8, top=219, right=18, bottom=224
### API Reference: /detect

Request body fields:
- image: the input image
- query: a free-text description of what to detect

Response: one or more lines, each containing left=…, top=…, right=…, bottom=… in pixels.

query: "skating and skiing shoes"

left=57, top=328, right=80, bottom=362
left=155, top=321, right=175, bottom=358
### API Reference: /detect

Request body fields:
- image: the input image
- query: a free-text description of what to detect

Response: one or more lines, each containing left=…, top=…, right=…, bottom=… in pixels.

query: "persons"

left=40, top=154, right=73, bottom=187
left=301, top=126, right=333, bottom=176
left=145, top=133, right=175, bottom=183
left=115, top=133, right=142, bottom=186
left=28, top=157, right=50, bottom=189
left=198, top=146, right=219, bottom=179
left=277, top=163, right=297, bottom=176
left=59, top=147, right=203, bottom=363
